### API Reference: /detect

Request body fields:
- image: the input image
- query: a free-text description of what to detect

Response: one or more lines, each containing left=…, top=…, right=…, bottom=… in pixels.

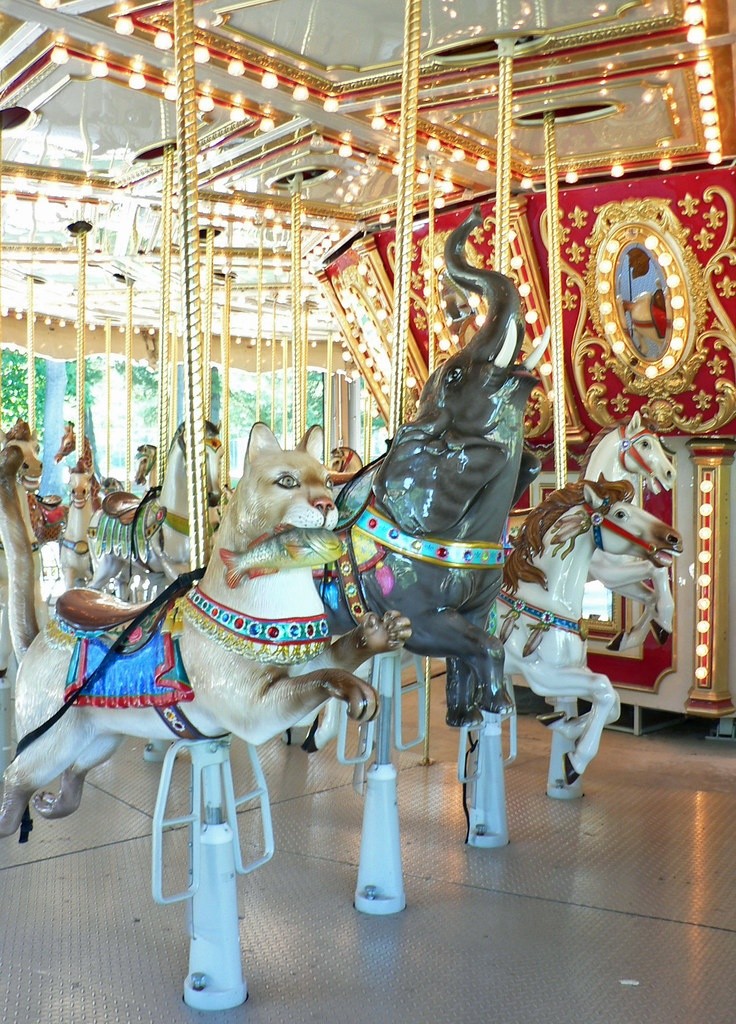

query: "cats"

left=1, top=425, right=380, bottom=842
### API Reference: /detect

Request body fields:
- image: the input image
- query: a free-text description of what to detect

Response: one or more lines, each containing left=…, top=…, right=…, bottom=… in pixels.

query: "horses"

left=0, top=415, right=227, bottom=668
left=488, top=410, right=680, bottom=788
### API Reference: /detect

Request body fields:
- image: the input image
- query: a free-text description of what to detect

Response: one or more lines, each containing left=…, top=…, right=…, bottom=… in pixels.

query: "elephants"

left=313, top=198, right=551, bottom=736
